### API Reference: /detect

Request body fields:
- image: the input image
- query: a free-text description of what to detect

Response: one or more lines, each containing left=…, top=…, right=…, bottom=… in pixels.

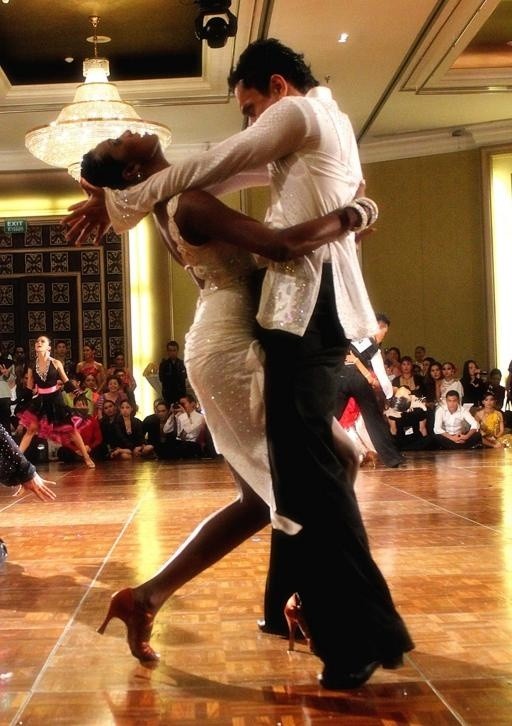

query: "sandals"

left=83, top=454, right=96, bottom=469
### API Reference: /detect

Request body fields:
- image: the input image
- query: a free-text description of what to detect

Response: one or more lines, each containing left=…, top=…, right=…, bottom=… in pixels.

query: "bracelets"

left=360, top=195, right=379, bottom=222
left=343, top=201, right=368, bottom=231
left=355, top=198, right=375, bottom=226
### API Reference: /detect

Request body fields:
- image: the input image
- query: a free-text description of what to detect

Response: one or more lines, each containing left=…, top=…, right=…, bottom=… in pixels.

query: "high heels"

left=95, top=587, right=160, bottom=662
left=284, top=593, right=315, bottom=653
left=364, top=451, right=377, bottom=468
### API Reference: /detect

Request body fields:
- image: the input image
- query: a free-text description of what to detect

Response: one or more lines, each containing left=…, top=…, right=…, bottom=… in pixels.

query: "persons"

left=57, top=36, right=415, bottom=692
left=82, top=127, right=378, bottom=660
left=2, top=314, right=214, bottom=467
left=0, top=424, right=57, bottom=563
left=339, top=315, right=512, bottom=466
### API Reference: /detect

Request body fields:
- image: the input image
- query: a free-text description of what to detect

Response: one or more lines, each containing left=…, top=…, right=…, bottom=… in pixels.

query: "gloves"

left=64, top=379, right=91, bottom=406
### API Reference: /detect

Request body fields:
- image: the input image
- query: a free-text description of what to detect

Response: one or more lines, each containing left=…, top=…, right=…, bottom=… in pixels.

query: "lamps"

left=22, top=12, right=173, bottom=183
left=194, top=0, right=238, bottom=48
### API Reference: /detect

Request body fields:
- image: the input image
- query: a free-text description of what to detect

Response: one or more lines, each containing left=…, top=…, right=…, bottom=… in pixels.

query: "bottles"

left=502, top=438, right=511, bottom=448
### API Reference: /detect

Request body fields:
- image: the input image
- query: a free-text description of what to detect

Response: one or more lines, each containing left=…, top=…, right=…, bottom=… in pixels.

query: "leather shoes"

left=315, top=647, right=404, bottom=691
left=257, top=619, right=304, bottom=640
